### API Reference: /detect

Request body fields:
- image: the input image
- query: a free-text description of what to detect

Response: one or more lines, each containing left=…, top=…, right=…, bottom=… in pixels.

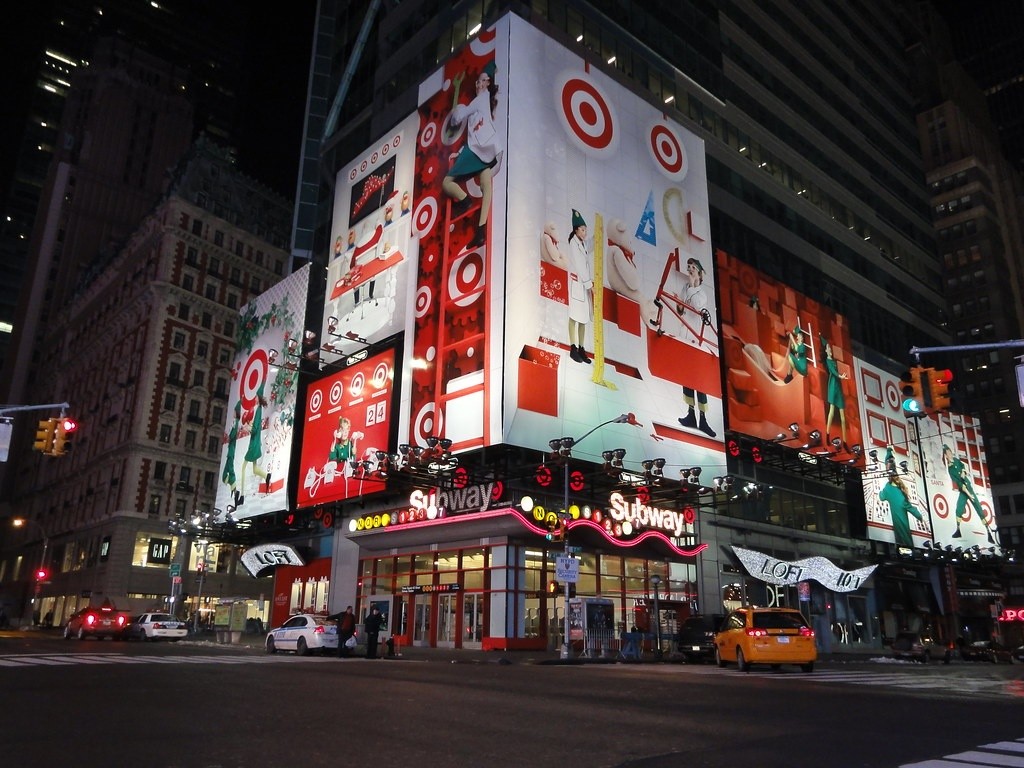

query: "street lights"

left=560, top=414, right=632, bottom=662
left=13, top=519, right=50, bottom=630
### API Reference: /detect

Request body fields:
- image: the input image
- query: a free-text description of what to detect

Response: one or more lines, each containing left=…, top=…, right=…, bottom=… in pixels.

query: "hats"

left=820, top=336, right=827, bottom=346
left=572, top=209, right=588, bottom=230
left=483, top=59, right=495, bottom=78
left=884, top=448, right=893, bottom=463
left=794, top=325, right=800, bottom=335
left=235, top=398, right=242, bottom=409
left=257, top=381, right=265, bottom=399
left=942, top=444, right=948, bottom=453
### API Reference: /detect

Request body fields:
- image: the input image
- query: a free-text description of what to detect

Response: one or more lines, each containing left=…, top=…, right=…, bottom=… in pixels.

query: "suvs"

left=677, top=604, right=818, bottom=672
left=893, top=631, right=943, bottom=664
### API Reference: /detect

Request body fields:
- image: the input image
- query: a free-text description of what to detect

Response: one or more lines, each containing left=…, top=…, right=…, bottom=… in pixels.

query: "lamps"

left=749, top=423, right=909, bottom=487
left=350, top=437, right=764, bottom=510
left=168, top=505, right=275, bottom=549
left=904, top=541, right=1017, bottom=566
left=266, top=316, right=374, bottom=377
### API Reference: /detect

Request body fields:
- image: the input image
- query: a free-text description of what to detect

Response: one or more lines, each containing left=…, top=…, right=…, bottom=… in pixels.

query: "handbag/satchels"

left=344, top=635, right=357, bottom=648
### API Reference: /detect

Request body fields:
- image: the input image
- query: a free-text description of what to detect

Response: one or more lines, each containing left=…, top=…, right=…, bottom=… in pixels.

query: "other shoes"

left=578, top=344, right=591, bottom=364
left=988, top=532, right=995, bottom=544
left=952, top=530, right=961, bottom=538
left=827, top=433, right=830, bottom=445
left=570, top=344, right=582, bottom=363
left=843, top=441, right=851, bottom=454
left=452, top=194, right=472, bottom=218
left=466, top=222, right=487, bottom=249
left=677, top=411, right=698, bottom=427
left=784, top=374, right=793, bottom=384
left=698, top=414, right=716, bottom=438
left=265, top=474, right=271, bottom=494
left=230, top=487, right=244, bottom=513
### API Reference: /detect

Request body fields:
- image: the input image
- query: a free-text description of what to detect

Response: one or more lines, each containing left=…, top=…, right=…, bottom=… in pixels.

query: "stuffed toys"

left=540, top=223, right=569, bottom=271
left=606, top=216, right=657, bottom=327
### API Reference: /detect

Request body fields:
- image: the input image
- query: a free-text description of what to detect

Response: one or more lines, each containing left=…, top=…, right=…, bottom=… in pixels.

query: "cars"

left=264, top=613, right=359, bottom=656
left=64, top=606, right=128, bottom=642
left=131, top=612, right=188, bottom=641
left=968, top=639, right=1014, bottom=662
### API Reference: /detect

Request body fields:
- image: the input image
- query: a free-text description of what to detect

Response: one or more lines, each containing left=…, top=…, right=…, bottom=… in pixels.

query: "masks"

left=373, top=610, right=378, bottom=614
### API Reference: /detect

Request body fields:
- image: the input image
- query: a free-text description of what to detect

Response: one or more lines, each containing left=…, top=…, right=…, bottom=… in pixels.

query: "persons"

left=772, top=331, right=807, bottom=384
left=237, top=382, right=271, bottom=505
left=325, top=606, right=355, bottom=658
left=442, top=60, right=504, bottom=249
left=673, top=257, right=716, bottom=438
left=44, top=609, right=53, bottom=630
left=568, top=209, right=593, bottom=363
left=879, top=447, right=923, bottom=547
left=329, top=418, right=357, bottom=463
left=222, top=399, right=242, bottom=511
left=364, top=607, right=383, bottom=659
left=820, top=336, right=851, bottom=454
left=942, top=444, right=995, bottom=544
left=33, top=609, right=40, bottom=627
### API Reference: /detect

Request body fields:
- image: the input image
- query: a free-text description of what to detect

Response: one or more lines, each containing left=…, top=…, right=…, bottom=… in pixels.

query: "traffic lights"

left=197, top=563, right=202, bottom=574
left=31, top=416, right=77, bottom=457
left=37, top=570, right=46, bottom=579
left=897, top=367, right=954, bottom=420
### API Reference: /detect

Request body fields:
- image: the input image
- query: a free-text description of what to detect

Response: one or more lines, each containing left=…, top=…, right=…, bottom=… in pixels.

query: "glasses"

left=475, top=76, right=490, bottom=85
left=688, top=264, right=700, bottom=272
